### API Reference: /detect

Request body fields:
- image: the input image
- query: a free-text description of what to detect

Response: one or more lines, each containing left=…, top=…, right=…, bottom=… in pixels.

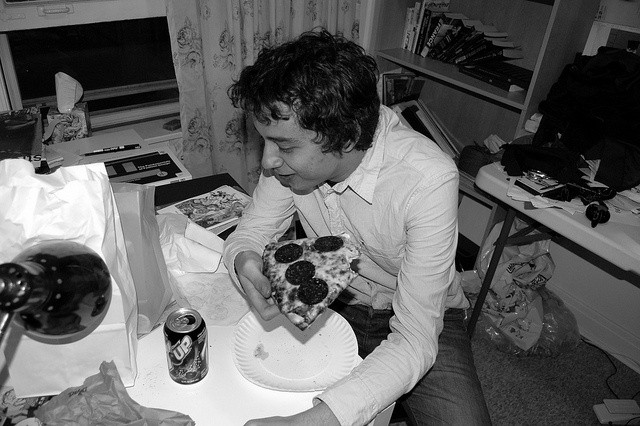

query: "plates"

left=232, top=306, right=359, bottom=392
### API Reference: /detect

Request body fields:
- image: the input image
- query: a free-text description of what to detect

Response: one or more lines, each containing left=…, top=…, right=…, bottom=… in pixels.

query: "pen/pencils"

left=81, top=142, right=142, bottom=157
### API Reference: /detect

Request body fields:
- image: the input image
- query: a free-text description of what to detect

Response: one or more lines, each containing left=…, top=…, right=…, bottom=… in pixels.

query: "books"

left=378, top=67, right=483, bottom=160
left=400, top=0, right=537, bottom=93
left=103, top=151, right=185, bottom=185
left=156, top=185, right=254, bottom=237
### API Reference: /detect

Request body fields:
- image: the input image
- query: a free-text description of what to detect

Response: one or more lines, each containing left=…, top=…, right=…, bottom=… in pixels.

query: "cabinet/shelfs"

left=369, top=0, right=601, bottom=274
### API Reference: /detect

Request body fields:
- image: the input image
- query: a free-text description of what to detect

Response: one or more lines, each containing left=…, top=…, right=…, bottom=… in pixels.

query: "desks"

left=44, top=128, right=396, bottom=426
left=467, top=146, right=639, bottom=342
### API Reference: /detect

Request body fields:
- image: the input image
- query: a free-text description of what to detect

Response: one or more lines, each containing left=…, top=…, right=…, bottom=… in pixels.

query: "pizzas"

left=261, top=230, right=362, bottom=333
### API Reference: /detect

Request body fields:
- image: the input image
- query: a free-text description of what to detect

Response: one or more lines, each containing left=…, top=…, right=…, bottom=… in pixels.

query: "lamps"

left=0, top=238, right=114, bottom=388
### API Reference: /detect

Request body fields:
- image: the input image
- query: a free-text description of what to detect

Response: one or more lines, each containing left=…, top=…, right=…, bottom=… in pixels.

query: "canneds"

left=163, top=308, right=209, bottom=384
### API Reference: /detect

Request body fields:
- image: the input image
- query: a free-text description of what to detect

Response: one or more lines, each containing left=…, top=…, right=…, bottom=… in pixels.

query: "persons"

left=221, top=25, right=496, bottom=425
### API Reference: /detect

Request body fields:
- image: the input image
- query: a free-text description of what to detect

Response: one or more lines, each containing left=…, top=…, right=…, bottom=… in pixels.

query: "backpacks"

left=534, top=46, right=640, bottom=192
